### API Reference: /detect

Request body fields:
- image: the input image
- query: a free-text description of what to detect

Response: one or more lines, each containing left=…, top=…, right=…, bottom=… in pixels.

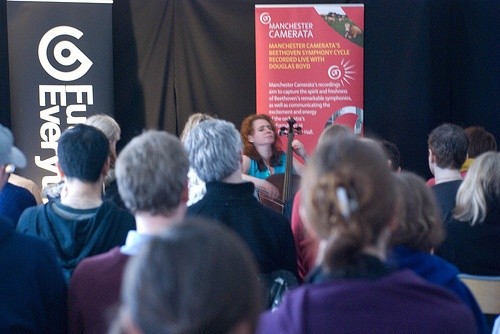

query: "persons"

left=0, top=115, right=500, bottom=334
left=254, top=134, right=479, bottom=334
left=67, top=130, right=191, bottom=334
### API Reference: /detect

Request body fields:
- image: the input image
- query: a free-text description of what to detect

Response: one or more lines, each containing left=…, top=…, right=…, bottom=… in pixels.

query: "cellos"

left=257, top=117, right=311, bottom=225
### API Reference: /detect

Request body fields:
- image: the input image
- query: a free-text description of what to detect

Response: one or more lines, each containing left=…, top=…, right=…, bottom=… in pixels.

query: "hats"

left=0, top=125, right=27, bottom=168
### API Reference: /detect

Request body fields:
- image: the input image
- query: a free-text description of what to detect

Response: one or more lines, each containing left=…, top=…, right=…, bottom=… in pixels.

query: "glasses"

left=5, top=165, right=15, bottom=173
